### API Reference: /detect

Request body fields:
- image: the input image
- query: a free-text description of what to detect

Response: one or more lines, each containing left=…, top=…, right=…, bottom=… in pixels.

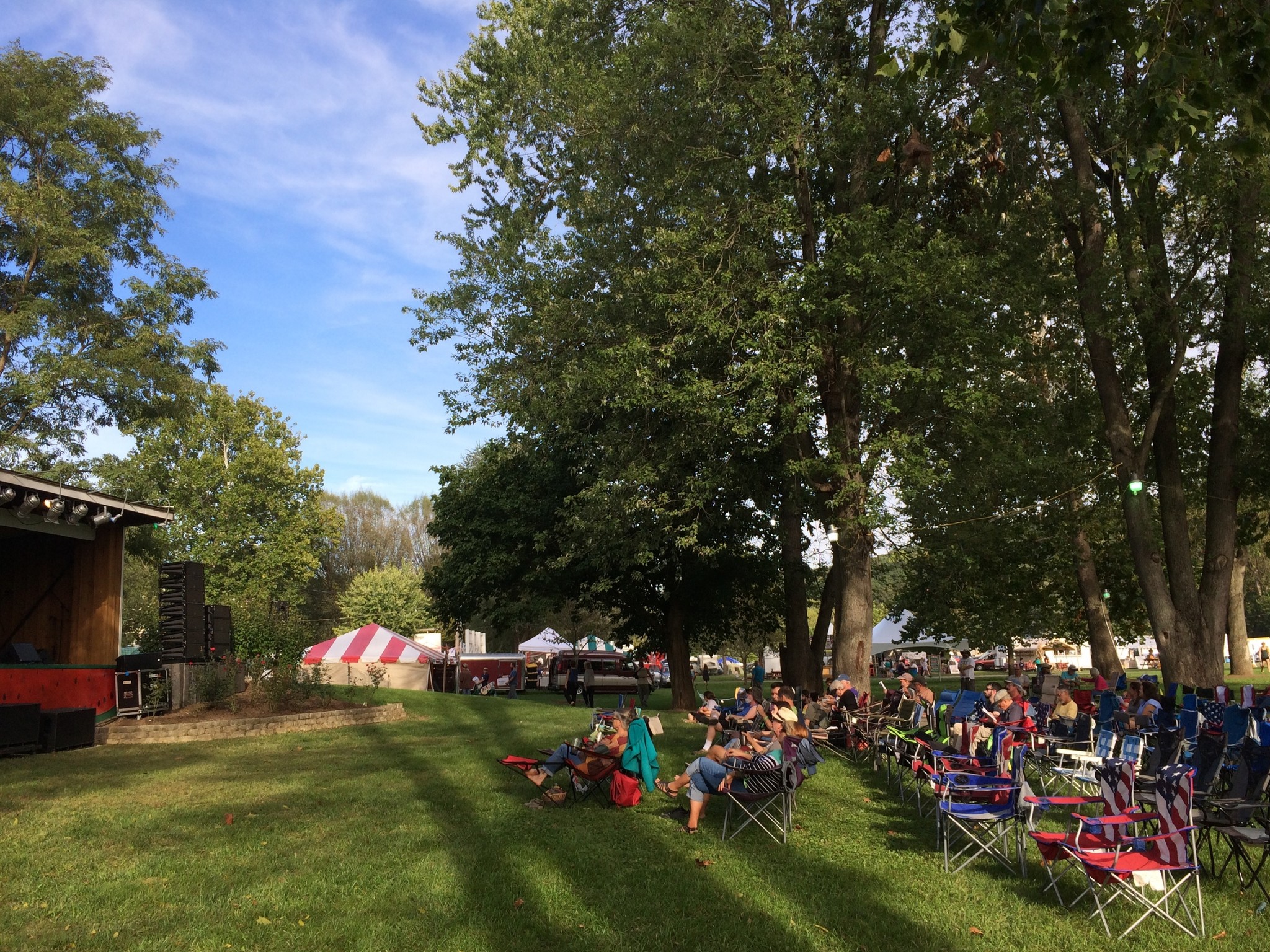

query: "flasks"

left=740, top=728, right=748, bottom=746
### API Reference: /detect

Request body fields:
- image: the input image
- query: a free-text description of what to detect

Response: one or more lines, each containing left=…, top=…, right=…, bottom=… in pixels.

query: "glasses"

left=826, top=683, right=830, bottom=686
left=746, top=692, right=752, bottom=694
left=914, top=683, right=917, bottom=686
left=781, top=728, right=788, bottom=736
left=986, top=688, right=993, bottom=691
left=807, top=696, right=810, bottom=698
left=802, top=695, right=804, bottom=697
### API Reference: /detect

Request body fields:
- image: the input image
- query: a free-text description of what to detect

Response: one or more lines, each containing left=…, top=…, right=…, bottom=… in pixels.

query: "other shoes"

left=700, top=716, right=719, bottom=725
left=682, top=719, right=696, bottom=723
left=685, top=762, right=692, bottom=769
left=692, top=748, right=707, bottom=755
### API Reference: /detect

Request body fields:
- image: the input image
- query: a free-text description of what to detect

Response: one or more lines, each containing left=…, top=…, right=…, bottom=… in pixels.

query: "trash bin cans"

left=908, top=667, right=917, bottom=676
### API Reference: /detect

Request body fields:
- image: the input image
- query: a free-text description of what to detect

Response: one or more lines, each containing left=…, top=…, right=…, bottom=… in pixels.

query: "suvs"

left=972, top=650, right=997, bottom=671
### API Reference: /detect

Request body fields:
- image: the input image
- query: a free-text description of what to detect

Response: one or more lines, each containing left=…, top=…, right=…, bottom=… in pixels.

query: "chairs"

left=496, top=672, right=1270, bottom=940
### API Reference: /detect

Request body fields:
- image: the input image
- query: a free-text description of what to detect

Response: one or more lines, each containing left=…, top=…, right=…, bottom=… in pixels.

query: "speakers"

left=205, top=604, right=233, bottom=662
left=0, top=703, right=40, bottom=746
left=157, top=560, right=205, bottom=662
left=40, top=706, right=97, bottom=751
left=0, top=642, right=42, bottom=665
left=117, top=651, right=160, bottom=673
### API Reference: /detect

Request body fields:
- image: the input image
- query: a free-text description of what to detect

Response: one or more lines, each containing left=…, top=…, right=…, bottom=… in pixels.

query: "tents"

left=717, top=657, right=738, bottom=664
left=262, top=622, right=456, bottom=691
left=870, top=604, right=969, bottom=657
left=518, top=627, right=638, bottom=658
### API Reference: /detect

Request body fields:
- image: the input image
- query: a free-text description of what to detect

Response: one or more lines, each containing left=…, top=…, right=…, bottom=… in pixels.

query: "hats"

left=463, top=663, right=467, bottom=668
left=801, top=690, right=809, bottom=697
left=1004, top=678, right=1021, bottom=685
left=772, top=707, right=798, bottom=722
left=836, top=674, right=850, bottom=682
left=745, top=686, right=763, bottom=703
left=910, top=676, right=927, bottom=686
left=897, top=673, right=914, bottom=681
left=960, top=649, right=971, bottom=656
left=993, top=689, right=1008, bottom=703
left=484, top=667, right=488, bottom=670
left=1069, top=665, right=1076, bottom=670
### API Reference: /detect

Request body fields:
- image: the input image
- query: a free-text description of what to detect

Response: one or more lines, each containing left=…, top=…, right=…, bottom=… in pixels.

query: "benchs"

left=1140, top=664, right=1158, bottom=665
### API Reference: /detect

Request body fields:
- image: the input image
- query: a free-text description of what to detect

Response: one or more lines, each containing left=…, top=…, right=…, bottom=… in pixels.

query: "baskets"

left=542, top=786, right=567, bottom=802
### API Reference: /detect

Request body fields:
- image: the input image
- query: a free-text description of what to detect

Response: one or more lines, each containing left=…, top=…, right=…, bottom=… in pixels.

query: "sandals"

left=654, top=778, right=679, bottom=798
left=674, top=825, right=699, bottom=834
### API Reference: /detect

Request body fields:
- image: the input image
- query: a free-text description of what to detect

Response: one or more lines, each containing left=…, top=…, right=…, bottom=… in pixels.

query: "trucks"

left=689, top=654, right=719, bottom=676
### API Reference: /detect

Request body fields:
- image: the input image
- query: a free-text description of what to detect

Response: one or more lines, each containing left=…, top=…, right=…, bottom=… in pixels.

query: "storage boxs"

left=115, top=651, right=162, bottom=673
left=115, top=668, right=169, bottom=716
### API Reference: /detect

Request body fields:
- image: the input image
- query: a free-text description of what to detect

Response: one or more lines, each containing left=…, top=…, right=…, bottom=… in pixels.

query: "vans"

left=647, top=665, right=671, bottom=688
left=549, top=650, right=661, bottom=695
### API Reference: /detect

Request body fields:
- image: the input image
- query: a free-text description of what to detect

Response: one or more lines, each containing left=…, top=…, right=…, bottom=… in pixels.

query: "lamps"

left=0, top=470, right=170, bottom=530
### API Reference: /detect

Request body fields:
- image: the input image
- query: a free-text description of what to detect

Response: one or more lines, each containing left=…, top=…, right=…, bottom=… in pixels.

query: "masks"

left=745, top=698, right=753, bottom=703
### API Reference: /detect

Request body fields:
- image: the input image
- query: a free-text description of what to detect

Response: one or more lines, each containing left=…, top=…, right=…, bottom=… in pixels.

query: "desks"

left=1143, top=659, right=1158, bottom=669
left=771, top=671, right=781, bottom=678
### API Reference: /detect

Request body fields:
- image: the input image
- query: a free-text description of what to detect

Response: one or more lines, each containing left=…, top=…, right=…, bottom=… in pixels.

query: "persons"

left=518, top=660, right=861, bottom=834
left=1258, top=642, right=1270, bottom=673
left=874, top=649, right=1164, bottom=742
left=473, top=667, right=489, bottom=694
left=958, top=649, right=975, bottom=692
left=504, top=662, right=517, bottom=699
left=459, top=663, right=472, bottom=695
